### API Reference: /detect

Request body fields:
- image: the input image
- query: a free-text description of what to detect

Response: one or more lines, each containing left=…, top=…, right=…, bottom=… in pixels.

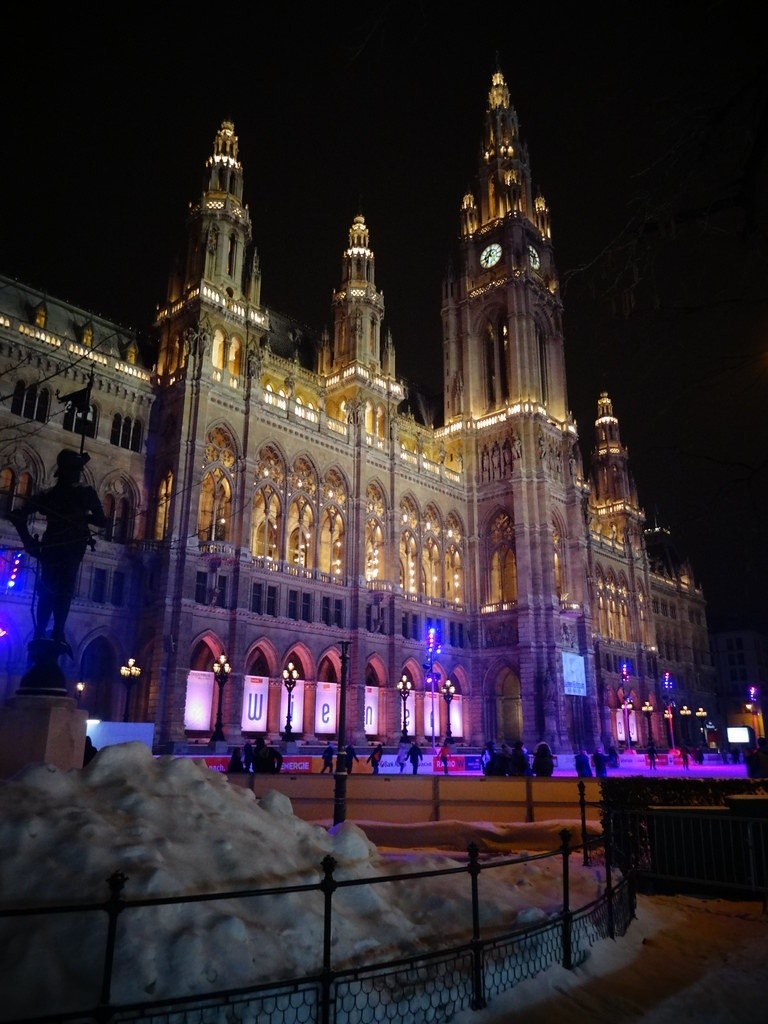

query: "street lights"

left=679, top=706, right=692, bottom=747
left=281, top=659, right=301, bottom=743
left=208, top=651, right=233, bottom=745
left=439, top=678, right=457, bottom=745
left=620, top=699, right=634, bottom=750
left=396, top=673, right=411, bottom=744
left=119, top=658, right=142, bottom=723
left=695, top=707, right=709, bottom=748
left=640, top=700, right=656, bottom=751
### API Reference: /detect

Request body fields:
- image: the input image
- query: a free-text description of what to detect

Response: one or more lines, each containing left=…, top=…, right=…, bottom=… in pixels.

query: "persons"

left=646, top=735, right=660, bottom=770
left=436, top=742, right=453, bottom=774
left=228, top=736, right=282, bottom=777
left=676, top=743, right=704, bottom=771
left=481, top=739, right=554, bottom=777
left=85, top=735, right=100, bottom=766
left=341, top=744, right=359, bottom=774
left=395, top=740, right=423, bottom=774
left=721, top=743, right=741, bottom=764
left=574, top=747, right=620, bottom=777
left=319, top=741, right=335, bottom=774
left=11, top=449, right=108, bottom=642
left=744, top=738, right=768, bottom=777
left=366, top=744, right=383, bottom=775
